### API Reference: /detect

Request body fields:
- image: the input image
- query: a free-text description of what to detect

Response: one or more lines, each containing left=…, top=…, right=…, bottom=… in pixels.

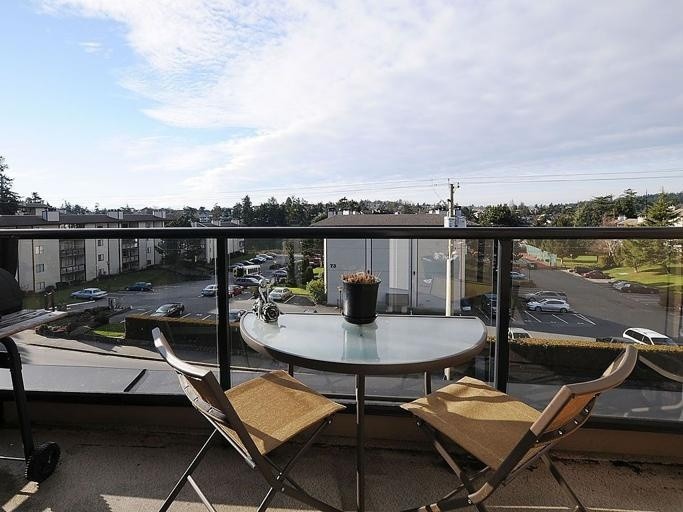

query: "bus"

left=233, top=265, right=261, bottom=277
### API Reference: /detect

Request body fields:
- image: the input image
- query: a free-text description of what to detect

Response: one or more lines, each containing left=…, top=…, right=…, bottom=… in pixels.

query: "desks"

left=240, top=312, right=488, bottom=512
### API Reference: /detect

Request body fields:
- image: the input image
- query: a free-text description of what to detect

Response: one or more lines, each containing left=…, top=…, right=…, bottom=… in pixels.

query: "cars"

left=267, top=286, right=293, bottom=301
left=69, top=288, right=108, bottom=301
left=279, top=268, right=285, bottom=270
left=508, top=327, right=534, bottom=339
left=527, top=262, right=537, bottom=270
left=607, top=279, right=659, bottom=295
left=595, top=336, right=637, bottom=344
left=510, top=267, right=527, bottom=280
left=200, top=284, right=216, bottom=297
left=124, top=281, right=152, bottom=292
left=228, top=252, right=277, bottom=271
left=149, top=302, right=185, bottom=317
left=522, top=289, right=571, bottom=313
left=227, top=285, right=243, bottom=298
left=274, top=270, right=287, bottom=276
left=234, top=274, right=271, bottom=287
left=574, top=265, right=609, bottom=279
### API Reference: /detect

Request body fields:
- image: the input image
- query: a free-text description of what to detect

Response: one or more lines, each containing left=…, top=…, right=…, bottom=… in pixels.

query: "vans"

left=622, top=327, right=679, bottom=346
left=481, top=293, right=513, bottom=319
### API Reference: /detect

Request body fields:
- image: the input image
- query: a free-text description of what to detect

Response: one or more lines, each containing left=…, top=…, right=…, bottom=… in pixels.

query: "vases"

left=340, top=273, right=382, bottom=325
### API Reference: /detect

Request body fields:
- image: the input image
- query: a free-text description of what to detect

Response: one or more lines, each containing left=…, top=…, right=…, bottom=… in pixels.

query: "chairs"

left=400, top=344, right=638, bottom=512
left=151, top=327, right=348, bottom=512
